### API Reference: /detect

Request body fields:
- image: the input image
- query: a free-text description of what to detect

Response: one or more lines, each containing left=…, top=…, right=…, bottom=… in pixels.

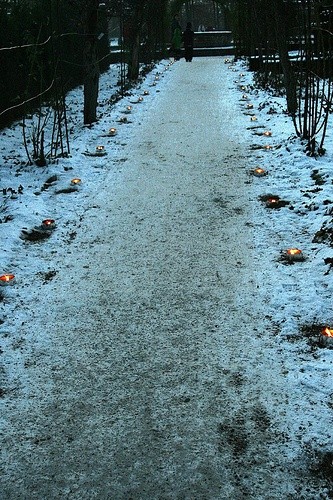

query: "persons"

left=180, top=22, right=195, bottom=62
